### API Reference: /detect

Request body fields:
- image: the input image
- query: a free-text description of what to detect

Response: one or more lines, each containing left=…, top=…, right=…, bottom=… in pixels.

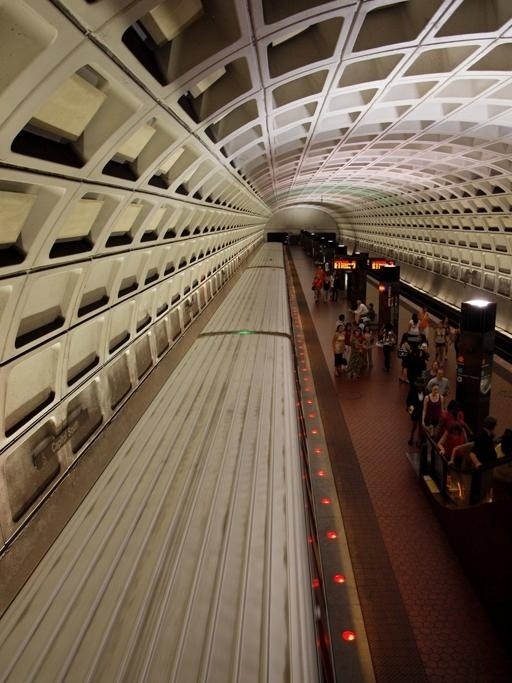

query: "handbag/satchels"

left=408, top=405, right=415, bottom=415
left=398, top=348, right=407, bottom=359
